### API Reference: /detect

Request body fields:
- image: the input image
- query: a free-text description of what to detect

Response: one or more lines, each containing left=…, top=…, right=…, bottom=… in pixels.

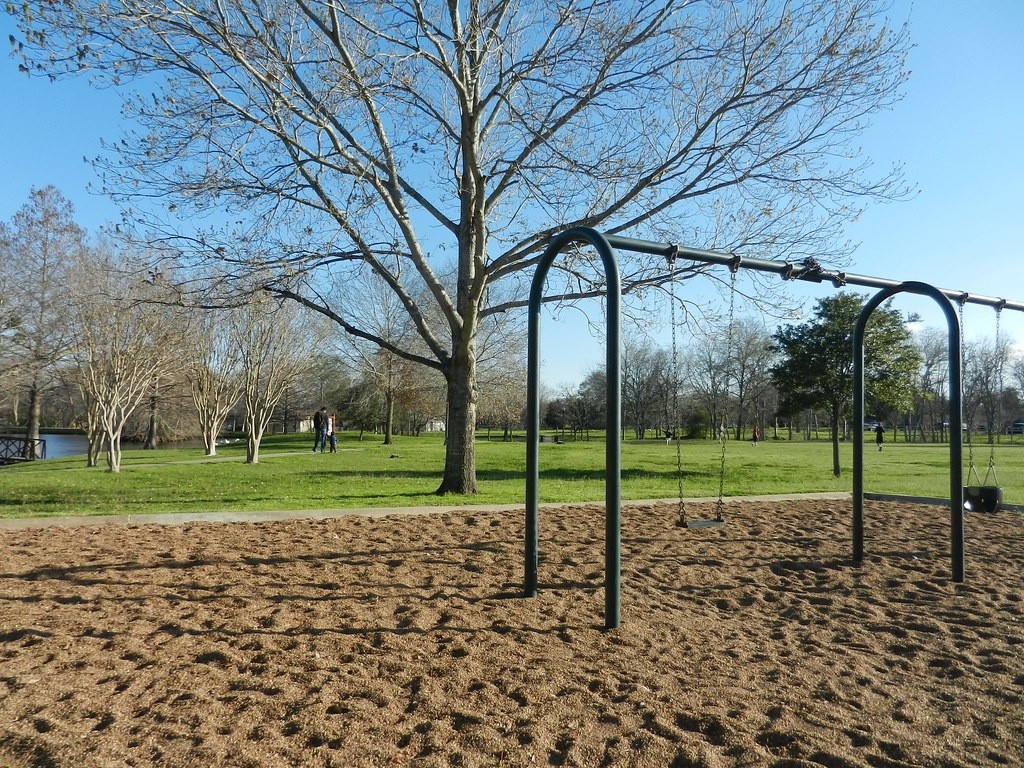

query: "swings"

left=958, top=301, right=1005, bottom=516
left=668, top=261, right=738, bottom=530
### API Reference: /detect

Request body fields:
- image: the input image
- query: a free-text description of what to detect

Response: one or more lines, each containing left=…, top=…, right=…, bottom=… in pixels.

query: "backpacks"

left=314, top=411, right=326, bottom=429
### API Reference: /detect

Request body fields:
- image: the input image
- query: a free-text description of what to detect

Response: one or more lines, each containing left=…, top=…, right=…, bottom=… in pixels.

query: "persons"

left=327, top=432, right=338, bottom=453
left=874, top=422, right=885, bottom=451
left=312, top=407, right=329, bottom=453
left=662, top=429, right=673, bottom=445
left=751, top=425, right=759, bottom=447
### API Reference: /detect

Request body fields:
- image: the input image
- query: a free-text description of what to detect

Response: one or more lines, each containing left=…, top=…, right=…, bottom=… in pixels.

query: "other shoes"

left=312, top=447, right=317, bottom=452
left=321, top=448, right=325, bottom=453
